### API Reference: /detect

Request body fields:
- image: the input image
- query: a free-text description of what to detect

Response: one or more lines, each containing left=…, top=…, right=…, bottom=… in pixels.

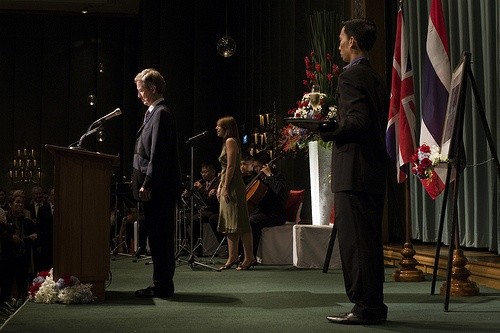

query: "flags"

left=385, top=0, right=457, bottom=200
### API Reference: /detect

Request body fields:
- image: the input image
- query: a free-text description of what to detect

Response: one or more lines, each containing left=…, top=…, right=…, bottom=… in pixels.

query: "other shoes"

left=135, top=280, right=174, bottom=298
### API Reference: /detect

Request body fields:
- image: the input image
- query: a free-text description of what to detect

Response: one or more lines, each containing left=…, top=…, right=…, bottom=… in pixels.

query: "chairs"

left=257, top=188, right=306, bottom=266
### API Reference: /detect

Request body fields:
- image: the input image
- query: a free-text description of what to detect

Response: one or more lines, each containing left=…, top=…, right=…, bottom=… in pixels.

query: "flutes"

left=181, top=178, right=204, bottom=199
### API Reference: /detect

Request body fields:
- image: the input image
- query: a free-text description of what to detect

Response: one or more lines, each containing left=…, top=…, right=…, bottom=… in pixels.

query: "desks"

left=291, top=225, right=343, bottom=270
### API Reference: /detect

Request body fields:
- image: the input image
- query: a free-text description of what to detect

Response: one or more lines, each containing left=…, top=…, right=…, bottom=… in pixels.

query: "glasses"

left=200, top=170, right=207, bottom=174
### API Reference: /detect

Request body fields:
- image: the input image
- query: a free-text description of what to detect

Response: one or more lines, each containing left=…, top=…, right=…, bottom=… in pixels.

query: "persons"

left=0, top=186, right=55, bottom=311
left=316, top=18, right=388, bottom=324
left=187, top=151, right=288, bottom=264
left=131, top=69, right=188, bottom=297
left=215, top=117, right=255, bottom=271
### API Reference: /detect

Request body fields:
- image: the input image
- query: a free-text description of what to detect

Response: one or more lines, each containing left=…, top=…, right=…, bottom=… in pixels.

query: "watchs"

left=269, top=173, right=273, bottom=176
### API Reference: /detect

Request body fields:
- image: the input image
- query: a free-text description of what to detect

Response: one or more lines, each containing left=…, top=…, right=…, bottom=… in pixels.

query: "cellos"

left=206, top=135, right=303, bottom=267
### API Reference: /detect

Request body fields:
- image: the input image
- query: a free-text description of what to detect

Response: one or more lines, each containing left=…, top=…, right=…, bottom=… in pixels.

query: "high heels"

left=236, top=258, right=257, bottom=271
left=218, top=257, right=240, bottom=270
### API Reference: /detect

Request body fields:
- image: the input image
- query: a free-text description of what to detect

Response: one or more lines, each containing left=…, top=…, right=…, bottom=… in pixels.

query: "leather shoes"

left=326, top=312, right=386, bottom=324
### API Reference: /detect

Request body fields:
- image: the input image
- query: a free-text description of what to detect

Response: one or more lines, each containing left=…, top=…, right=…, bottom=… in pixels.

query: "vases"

left=307, top=137, right=335, bottom=225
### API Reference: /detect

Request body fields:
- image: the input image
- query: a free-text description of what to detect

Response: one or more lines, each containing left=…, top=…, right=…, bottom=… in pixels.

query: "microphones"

left=93, top=108, right=121, bottom=125
left=186, top=131, right=209, bottom=144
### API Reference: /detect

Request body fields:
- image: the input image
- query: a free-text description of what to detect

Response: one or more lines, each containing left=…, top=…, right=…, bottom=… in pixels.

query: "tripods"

left=175, top=141, right=223, bottom=270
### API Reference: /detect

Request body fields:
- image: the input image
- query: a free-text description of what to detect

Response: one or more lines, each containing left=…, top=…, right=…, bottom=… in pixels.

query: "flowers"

left=27, top=270, right=98, bottom=305
left=410, top=144, right=452, bottom=180
left=272, top=9, right=345, bottom=158
